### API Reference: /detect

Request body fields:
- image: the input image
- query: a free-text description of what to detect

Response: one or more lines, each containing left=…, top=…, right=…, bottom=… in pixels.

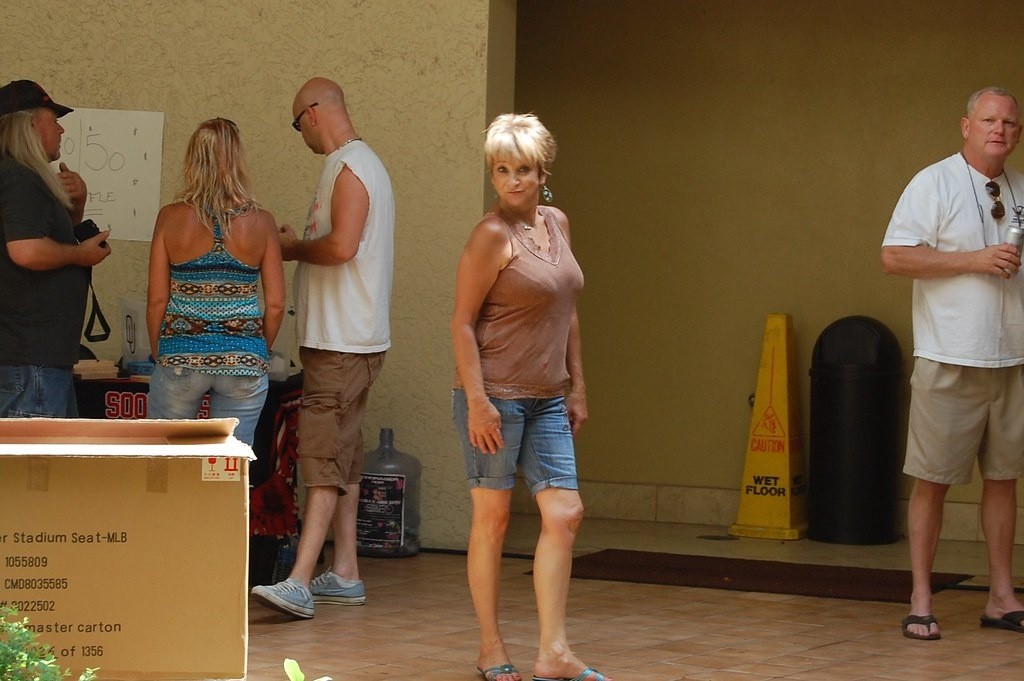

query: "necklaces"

left=962, top=151, right=1021, bottom=247
left=337, top=139, right=361, bottom=149
left=518, top=221, right=531, bottom=229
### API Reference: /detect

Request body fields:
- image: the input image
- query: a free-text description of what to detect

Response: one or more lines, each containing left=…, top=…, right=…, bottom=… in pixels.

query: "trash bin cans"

left=809, top=315, right=903, bottom=545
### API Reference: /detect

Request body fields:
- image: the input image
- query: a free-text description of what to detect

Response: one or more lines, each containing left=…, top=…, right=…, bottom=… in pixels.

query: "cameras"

left=73, top=219, right=106, bottom=248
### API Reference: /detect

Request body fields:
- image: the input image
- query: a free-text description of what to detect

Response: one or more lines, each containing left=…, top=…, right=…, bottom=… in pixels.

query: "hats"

left=0, top=80, right=73, bottom=118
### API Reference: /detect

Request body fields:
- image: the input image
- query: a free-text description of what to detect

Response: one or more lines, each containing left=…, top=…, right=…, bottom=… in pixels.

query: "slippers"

left=901, top=614, right=939, bottom=639
left=980, top=610, right=1024, bottom=633
left=477, top=661, right=521, bottom=681
left=534, top=666, right=613, bottom=681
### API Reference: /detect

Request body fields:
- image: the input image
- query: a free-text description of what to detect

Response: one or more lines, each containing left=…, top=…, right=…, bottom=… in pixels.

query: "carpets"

left=521, top=548, right=975, bottom=604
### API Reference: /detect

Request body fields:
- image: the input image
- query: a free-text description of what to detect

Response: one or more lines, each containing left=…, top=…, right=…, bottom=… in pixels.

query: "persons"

left=249, top=78, right=393, bottom=618
left=146, top=117, right=286, bottom=448
left=451, top=112, right=610, bottom=681
left=0, top=79, right=112, bottom=417
left=878, top=89, right=1024, bottom=641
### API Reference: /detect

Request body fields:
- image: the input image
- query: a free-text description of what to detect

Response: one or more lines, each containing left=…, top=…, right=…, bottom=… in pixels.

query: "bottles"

left=356, top=428, right=422, bottom=559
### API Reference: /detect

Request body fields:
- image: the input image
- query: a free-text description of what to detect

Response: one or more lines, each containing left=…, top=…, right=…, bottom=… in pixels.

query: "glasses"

left=292, top=102, right=319, bottom=132
left=986, top=181, right=1005, bottom=220
left=209, top=118, right=240, bottom=138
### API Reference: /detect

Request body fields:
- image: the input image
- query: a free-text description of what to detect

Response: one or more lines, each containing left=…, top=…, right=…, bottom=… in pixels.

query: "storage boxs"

left=128, top=362, right=154, bottom=376
left=122, top=294, right=153, bottom=368
left=260, top=299, right=295, bottom=382
left=0, top=417, right=258, bottom=681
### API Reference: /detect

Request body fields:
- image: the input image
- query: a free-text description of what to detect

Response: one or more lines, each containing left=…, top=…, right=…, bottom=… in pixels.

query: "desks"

left=74, top=378, right=301, bottom=536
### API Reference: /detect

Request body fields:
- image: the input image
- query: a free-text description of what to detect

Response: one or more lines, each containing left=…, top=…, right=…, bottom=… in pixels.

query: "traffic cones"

left=726, top=312, right=811, bottom=540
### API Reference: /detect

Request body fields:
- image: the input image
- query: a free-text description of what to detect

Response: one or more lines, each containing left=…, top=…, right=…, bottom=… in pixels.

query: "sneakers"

left=251, top=578, right=315, bottom=618
left=309, top=565, right=365, bottom=606
